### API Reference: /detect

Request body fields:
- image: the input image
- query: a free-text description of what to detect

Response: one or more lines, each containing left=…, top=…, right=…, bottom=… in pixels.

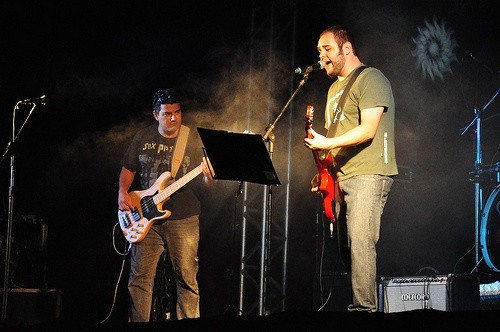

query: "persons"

left=303, top=25, right=399, bottom=313
left=117, top=89, right=217, bottom=322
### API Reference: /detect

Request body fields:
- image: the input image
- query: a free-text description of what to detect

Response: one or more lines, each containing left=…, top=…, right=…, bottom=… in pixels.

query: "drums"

left=478, top=184, right=500, bottom=273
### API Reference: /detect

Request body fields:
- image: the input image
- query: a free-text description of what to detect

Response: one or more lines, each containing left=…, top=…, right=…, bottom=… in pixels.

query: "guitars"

left=303, top=105, right=336, bottom=223
left=117, top=129, right=257, bottom=244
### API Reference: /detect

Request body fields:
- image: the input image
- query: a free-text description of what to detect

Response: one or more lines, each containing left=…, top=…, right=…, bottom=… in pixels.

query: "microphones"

left=295, top=61, right=326, bottom=74
left=18, top=95, right=48, bottom=105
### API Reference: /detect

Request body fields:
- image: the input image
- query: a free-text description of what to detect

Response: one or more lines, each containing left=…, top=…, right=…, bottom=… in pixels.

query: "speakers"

left=384, top=273, right=456, bottom=313
